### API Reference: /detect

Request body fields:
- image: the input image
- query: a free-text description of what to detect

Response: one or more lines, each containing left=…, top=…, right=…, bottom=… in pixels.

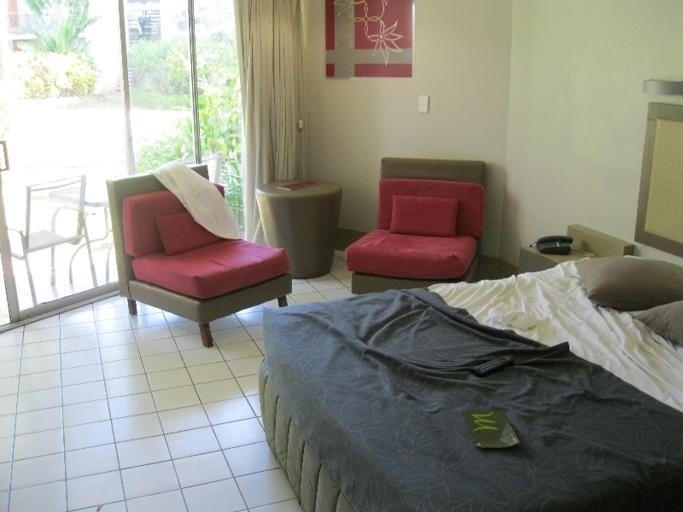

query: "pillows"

left=155, top=211, right=222, bottom=256
left=390, top=196, right=459, bottom=236
left=575, top=257, right=683, bottom=311
left=633, top=300, right=682, bottom=347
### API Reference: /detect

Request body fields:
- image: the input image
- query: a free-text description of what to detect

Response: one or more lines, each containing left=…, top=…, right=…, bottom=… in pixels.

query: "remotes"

left=474, top=354, right=515, bottom=378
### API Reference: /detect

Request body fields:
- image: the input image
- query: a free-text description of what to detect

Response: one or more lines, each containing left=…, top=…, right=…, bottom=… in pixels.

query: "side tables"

left=255, top=180, right=342, bottom=280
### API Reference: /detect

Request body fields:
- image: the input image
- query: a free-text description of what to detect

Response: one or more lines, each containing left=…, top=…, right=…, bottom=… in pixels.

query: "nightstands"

left=518, top=243, right=598, bottom=275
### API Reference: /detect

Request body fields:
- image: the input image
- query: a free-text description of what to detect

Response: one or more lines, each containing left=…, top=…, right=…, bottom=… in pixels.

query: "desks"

left=49, top=189, right=113, bottom=285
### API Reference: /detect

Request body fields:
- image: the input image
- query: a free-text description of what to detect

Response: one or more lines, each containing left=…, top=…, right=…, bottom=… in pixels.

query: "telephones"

left=530, top=236, right=573, bottom=255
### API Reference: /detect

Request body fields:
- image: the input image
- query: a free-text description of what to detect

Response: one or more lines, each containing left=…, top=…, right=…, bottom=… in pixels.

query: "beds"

left=258, top=254, right=683, bottom=510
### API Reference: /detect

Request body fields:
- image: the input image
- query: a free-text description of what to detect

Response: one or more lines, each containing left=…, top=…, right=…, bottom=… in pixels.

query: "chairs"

left=345, top=157, right=485, bottom=295
left=106, top=163, right=291, bottom=348
left=6, top=175, right=98, bottom=307
